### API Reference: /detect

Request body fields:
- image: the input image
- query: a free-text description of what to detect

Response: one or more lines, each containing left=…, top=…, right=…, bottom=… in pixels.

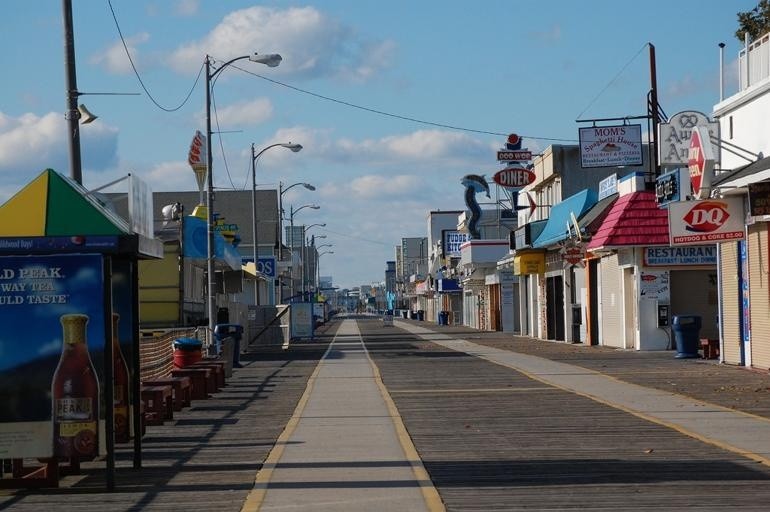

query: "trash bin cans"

left=438, top=311, right=449, bottom=325
left=418, top=310, right=424, bottom=320
left=672, top=313, right=702, bottom=359
left=172, top=338, right=202, bottom=369
left=403, top=312, right=407, bottom=318
left=214, top=325, right=244, bottom=368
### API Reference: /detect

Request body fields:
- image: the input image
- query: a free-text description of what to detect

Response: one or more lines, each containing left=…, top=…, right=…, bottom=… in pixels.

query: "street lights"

left=204, top=53, right=334, bottom=345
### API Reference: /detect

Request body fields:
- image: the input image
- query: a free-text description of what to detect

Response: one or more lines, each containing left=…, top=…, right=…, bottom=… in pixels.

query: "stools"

left=140, top=359, right=230, bottom=427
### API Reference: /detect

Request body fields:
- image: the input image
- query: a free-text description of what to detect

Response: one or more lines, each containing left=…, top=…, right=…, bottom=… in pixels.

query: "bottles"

left=112, top=313, right=129, bottom=443
left=54, top=314, right=100, bottom=461
